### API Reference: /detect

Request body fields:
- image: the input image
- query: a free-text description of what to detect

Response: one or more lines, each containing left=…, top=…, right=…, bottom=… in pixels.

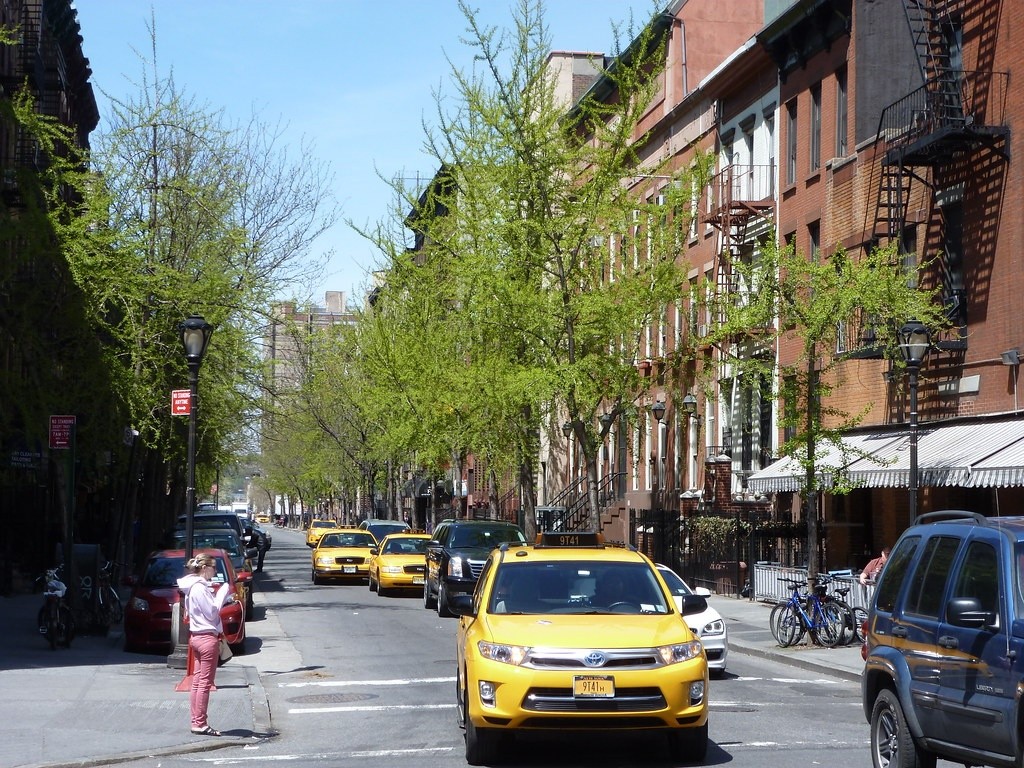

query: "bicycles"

left=33, top=562, right=78, bottom=651
left=96, top=559, right=129, bottom=632
left=770, top=577, right=869, bottom=649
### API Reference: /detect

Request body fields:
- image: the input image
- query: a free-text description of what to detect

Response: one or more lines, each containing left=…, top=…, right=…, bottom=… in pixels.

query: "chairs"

left=352, top=535, right=367, bottom=542
left=326, top=534, right=343, bottom=545
left=391, top=542, right=401, bottom=551
left=214, top=541, right=232, bottom=552
left=506, top=576, right=541, bottom=608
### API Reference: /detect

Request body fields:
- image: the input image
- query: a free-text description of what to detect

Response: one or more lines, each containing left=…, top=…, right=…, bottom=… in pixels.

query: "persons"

left=403, top=511, right=412, bottom=529
left=283, top=515, right=288, bottom=527
left=860, top=547, right=891, bottom=594
left=245, top=527, right=268, bottom=573
left=177, top=554, right=231, bottom=736
left=274, top=514, right=280, bottom=520
left=593, top=571, right=630, bottom=606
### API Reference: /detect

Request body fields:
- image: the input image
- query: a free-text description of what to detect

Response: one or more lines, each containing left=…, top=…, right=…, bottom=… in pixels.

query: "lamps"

left=1000, top=349, right=1020, bottom=366
left=599, top=413, right=617, bottom=439
left=651, top=398, right=670, bottom=431
left=562, top=421, right=574, bottom=442
left=682, top=391, right=702, bottom=427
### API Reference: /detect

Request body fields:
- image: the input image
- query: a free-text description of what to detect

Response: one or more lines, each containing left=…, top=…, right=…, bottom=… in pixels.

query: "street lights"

left=895, top=316, right=934, bottom=526
left=167, top=311, right=215, bottom=670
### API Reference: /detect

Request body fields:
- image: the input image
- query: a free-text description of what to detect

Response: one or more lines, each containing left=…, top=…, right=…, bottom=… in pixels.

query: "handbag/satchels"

left=216, top=640, right=233, bottom=666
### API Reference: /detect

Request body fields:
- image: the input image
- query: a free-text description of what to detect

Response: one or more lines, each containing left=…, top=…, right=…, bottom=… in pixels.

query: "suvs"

left=170, top=502, right=271, bottom=623
left=423, top=517, right=530, bottom=618
left=861, top=509, right=1024, bottom=768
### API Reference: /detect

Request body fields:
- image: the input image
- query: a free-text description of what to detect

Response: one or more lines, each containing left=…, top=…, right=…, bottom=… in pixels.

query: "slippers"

left=192, top=722, right=220, bottom=736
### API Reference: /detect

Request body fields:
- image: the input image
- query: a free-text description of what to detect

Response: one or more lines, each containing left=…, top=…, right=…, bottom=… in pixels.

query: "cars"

left=646, top=563, right=728, bottom=680
left=123, top=548, right=254, bottom=656
left=309, top=528, right=381, bottom=585
left=352, top=518, right=419, bottom=552
left=304, top=518, right=339, bottom=547
left=368, top=533, right=433, bottom=597
left=446, top=530, right=709, bottom=766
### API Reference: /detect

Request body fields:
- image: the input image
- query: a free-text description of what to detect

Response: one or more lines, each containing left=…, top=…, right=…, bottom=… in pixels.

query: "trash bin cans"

left=53, top=545, right=107, bottom=638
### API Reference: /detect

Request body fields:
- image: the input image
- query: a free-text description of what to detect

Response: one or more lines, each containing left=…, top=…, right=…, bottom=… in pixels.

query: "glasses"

left=204, top=564, right=216, bottom=569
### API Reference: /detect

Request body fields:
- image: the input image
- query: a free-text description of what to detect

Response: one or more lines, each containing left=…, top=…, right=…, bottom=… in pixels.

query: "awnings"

left=415, top=478, right=430, bottom=497
left=967, top=439, right=1024, bottom=487
left=838, top=416, right=1024, bottom=489
left=401, top=477, right=420, bottom=498
left=747, top=435, right=902, bottom=492
